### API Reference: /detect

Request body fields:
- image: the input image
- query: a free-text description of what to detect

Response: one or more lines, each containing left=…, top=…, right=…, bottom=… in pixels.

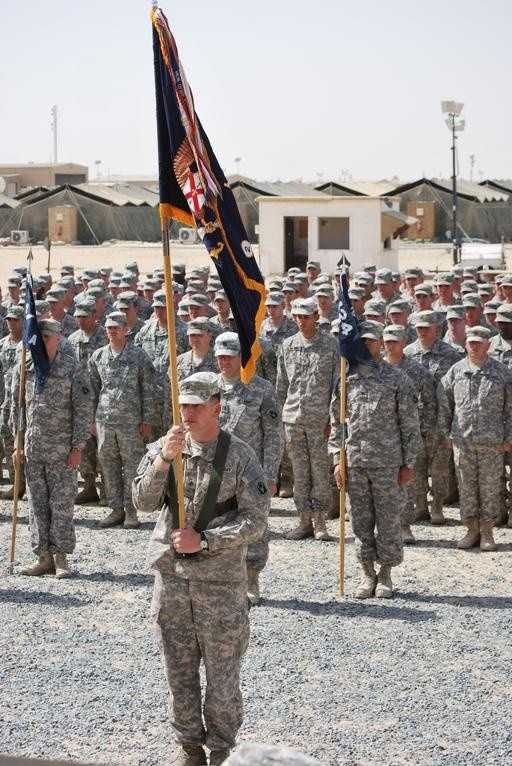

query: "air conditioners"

left=178, top=227, right=198, bottom=242
left=11, top=230, right=30, bottom=244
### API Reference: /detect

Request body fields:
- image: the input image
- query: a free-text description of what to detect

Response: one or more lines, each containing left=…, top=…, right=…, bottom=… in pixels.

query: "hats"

left=176, top=369, right=223, bottom=407
left=349, top=265, right=511, bottom=345
left=3, top=261, right=241, bottom=357
left=266, top=260, right=341, bottom=317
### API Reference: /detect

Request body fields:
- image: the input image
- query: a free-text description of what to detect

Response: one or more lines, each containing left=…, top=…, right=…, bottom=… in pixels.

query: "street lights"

left=234, top=156, right=242, bottom=173
left=439, top=96, right=471, bottom=266
left=468, top=151, right=475, bottom=181
left=93, top=158, right=102, bottom=177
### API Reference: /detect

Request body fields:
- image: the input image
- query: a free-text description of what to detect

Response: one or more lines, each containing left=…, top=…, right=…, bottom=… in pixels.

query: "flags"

left=150, top=6, right=266, bottom=386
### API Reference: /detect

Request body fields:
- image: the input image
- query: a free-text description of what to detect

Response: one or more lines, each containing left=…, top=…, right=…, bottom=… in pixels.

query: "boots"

left=100, top=508, right=126, bottom=528
left=95, top=481, right=108, bottom=505
left=52, top=552, right=72, bottom=578
left=167, top=747, right=208, bottom=766
left=3, top=482, right=25, bottom=500
left=208, top=750, right=231, bottom=766
left=22, top=552, right=55, bottom=577
left=75, top=477, right=98, bottom=504
left=355, top=560, right=379, bottom=600
left=376, top=560, right=395, bottom=598
left=275, top=477, right=512, bottom=549
left=243, top=567, right=263, bottom=605
left=124, top=509, right=139, bottom=529
